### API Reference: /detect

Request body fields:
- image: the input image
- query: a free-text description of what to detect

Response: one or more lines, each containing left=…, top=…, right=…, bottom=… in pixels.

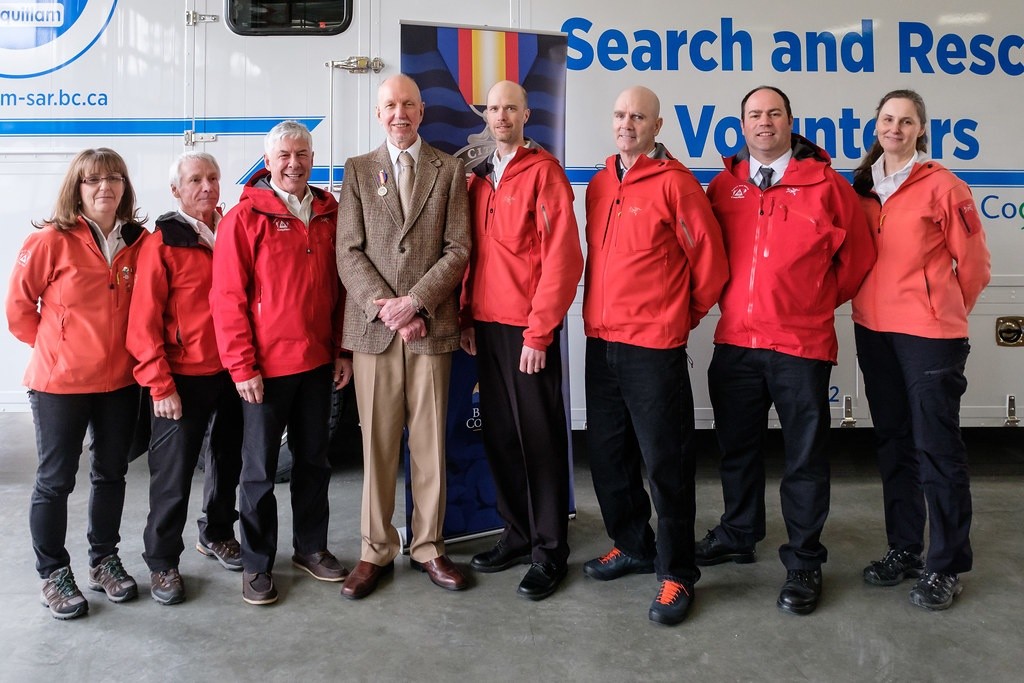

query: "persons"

left=335, top=73, right=473, bottom=600
left=693, top=86, right=877, bottom=615
left=852, top=89, right=992, bottom=611
left=124, top=152, right=244, bottom=605
left=208, top=120, right=353, bottom=605
left=458, top=79, right=584, bottom=602
left=582, top=87, right=729, bottom=626
left=6, top=148, right=151, bottom=619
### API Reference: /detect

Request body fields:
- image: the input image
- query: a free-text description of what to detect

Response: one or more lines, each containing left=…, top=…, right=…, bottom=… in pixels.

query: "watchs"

left=410, top=293, right=420, bottom=313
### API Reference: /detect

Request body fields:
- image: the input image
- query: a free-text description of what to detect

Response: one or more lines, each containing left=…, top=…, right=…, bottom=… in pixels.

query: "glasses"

left=79, top=174, right=126, bottom=185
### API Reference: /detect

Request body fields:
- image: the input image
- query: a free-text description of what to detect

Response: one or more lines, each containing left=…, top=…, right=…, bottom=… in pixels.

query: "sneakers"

left=88, top=553, right=138, bottom=603
left=150, top=566, right=186, bottom=605
left=195, top=534, right=243, bottom=570
left=41, top=565, right=90, bottom=620
left=649, top=577, right=695, bottom=625
left=862, top=547, right=926, bottom=587
left=582, top=547, right=659, bottom=581
left=908, top=562, right=964, bottom=611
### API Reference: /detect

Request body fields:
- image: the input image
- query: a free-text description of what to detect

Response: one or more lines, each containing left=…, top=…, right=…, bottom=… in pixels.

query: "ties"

left=758, top=167, right=774, bottom=191
left=398, top=151, right=416, bottom=223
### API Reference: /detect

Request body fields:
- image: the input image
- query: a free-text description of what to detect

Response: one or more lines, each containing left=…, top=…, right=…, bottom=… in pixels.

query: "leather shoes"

left=339, top=559, right=395, bottom=600
left=409, top=554, right=469, bottom=591
left=776, top=566, right=822, bottom=615
left=292, top=545, right=350, bottom=582
left=516, top=560, right=568, bottom=600
left=470, top=540, right=532, bottom=573
left=694, top=524, right=759, bottom=567
left=242, top=567, right=278, bottom=605
